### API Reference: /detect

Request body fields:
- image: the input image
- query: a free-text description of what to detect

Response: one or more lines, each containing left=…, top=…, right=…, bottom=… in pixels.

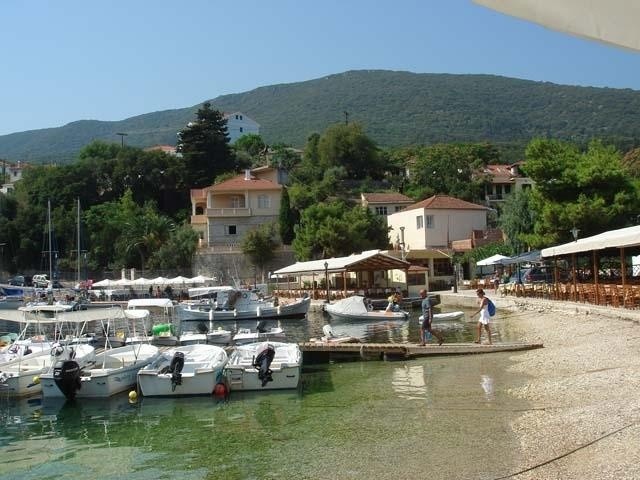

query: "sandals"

left=416, top=342, right=426, bottom=346
left=437, top=337, right=446, bottom=346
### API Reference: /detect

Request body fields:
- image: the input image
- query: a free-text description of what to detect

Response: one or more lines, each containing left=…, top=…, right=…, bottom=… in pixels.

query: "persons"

left=302, top=278, right=332, bottom=289
left=415, top=289, right=446, bottom=347
left=386, top=291, right=402, bottom=312
left=365, top=299, right=374, bottom=312
left=421, top=358, right=451, bottom=421
left=469, top=288, right=492, bottom=345
left=475, top=351, right=500, bottom=409
left=349, top=274, right=380, bottom=289
left=166, top=284, right=172, bottom=299
left=149, top=285, right=154, bottom=297
left=156, top=286, right=161, bottom=298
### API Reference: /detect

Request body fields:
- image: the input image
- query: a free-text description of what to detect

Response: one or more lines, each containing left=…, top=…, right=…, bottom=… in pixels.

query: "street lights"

left=324, top=262, right=330, bottom=303
left=570, top=226, right=582, bottom=277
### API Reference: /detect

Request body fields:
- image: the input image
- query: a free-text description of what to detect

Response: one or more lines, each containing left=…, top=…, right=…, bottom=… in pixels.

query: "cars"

left=509, top=265, right=570, bottom=284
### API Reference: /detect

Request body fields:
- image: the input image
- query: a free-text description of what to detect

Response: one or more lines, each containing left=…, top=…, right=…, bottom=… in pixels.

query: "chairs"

left=494, top=278, right=640, bottom=310
left=273, top=287, right=407, bottom=301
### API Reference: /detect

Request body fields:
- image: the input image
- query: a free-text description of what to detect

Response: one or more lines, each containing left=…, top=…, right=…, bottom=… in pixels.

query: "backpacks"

left=483, top=297, right=495, bottom=316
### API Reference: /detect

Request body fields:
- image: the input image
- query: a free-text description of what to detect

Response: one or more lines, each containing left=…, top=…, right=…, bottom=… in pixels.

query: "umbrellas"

left=114, top=276, right=136, bottom=301
left=91, top=278, right=118, bottom=301
left=129, top=276, right=154, bottom=299
left=174, top=275, right=195, bottom=300
left=192, top=275, right=215, bottom=284
left=152, top=276, right=175, bottom=298
